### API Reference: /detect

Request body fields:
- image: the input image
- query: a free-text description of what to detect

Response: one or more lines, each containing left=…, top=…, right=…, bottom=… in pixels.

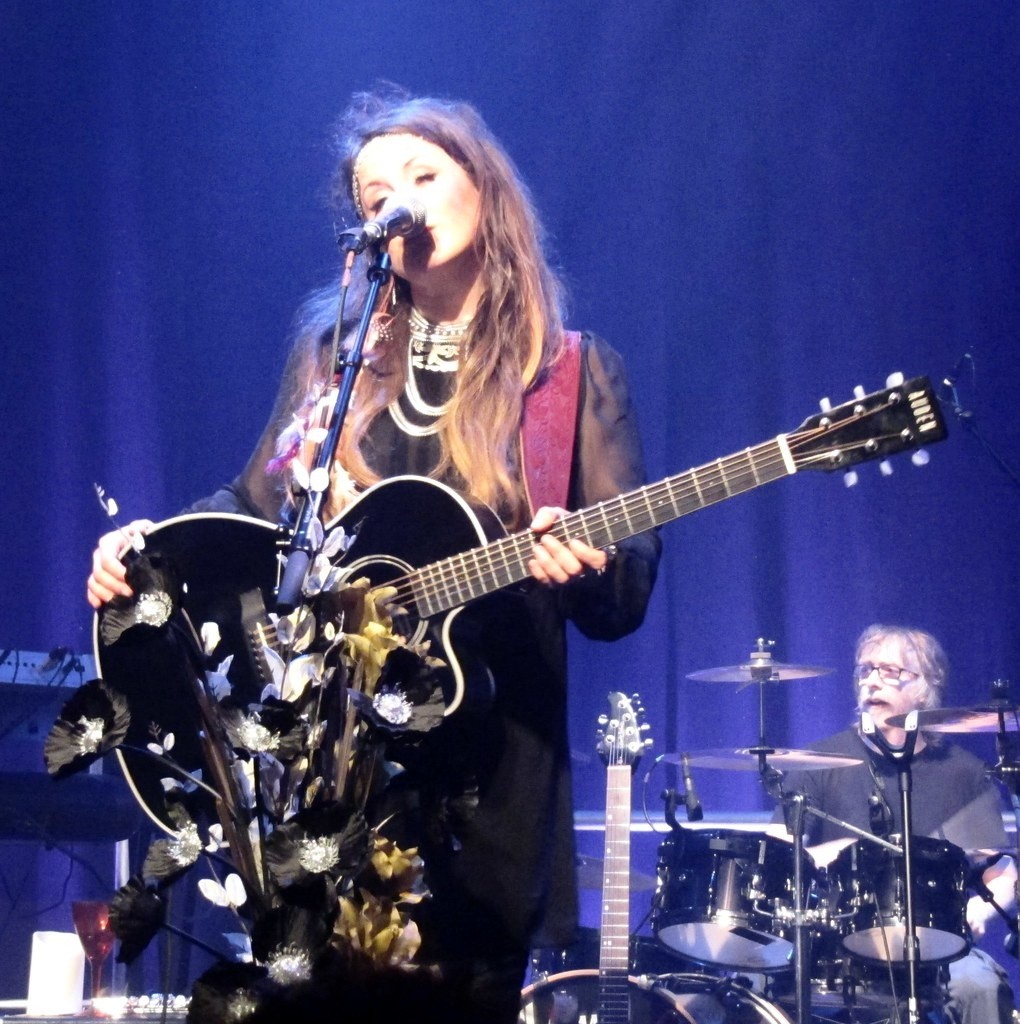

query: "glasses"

left=851, top=662, right=923, bottom=683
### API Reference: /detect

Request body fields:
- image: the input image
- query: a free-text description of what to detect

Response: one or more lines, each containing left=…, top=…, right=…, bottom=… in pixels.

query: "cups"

left=26, top=931, right=85, bottom=1017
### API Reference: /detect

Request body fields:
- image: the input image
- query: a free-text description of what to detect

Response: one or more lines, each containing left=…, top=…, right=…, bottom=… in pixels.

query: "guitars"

left=595, top=686, right=644, bottom=1024
left=91, top=369, right=951, bottom=856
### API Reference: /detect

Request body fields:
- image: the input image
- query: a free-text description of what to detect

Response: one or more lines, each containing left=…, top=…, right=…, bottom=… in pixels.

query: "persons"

left=83, top=93, right=661, bottom=1024
left=772, top=624, right=1020, bottom=1023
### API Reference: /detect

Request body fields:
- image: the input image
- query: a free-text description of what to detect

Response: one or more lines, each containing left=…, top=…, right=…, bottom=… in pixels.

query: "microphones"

left=680, top=752, right=704, bottom=821
left=347, top=196, right=427, bottom=255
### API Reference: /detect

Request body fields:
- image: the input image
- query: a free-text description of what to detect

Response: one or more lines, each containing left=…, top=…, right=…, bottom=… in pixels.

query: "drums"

left=651, top=824, right=816, bottom=973
left=827, top=833, right=975, bottom=972
left=515, top=968, right=796, bottom=1024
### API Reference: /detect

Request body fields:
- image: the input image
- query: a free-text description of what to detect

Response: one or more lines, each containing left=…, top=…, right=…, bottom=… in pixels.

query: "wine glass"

left=69, top=899, right=117, bottom=1020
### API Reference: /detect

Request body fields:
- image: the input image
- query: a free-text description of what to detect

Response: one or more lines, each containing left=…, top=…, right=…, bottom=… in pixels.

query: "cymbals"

left=685, top=652, right=838, bottom=687
left=654, top=745, right=867, bottom=772
left=883, top=694, right=1020, bottom=734
